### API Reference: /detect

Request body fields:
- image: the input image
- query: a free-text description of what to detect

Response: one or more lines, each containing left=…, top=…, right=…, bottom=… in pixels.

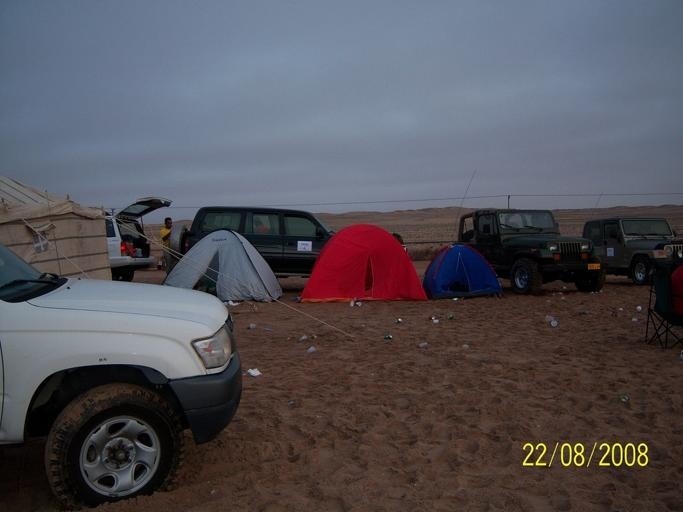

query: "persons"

left=159, top=217, right=173, bottom=273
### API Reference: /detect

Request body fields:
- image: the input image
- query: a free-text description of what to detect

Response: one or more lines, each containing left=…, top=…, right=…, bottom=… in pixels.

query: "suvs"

left=458, top=208, right=683, bottom=295
left=169, top=196, right=407, bottom=281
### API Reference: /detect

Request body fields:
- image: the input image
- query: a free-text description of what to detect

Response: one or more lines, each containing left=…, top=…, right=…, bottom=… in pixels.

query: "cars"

left=0, top=239, right=243, bottom=512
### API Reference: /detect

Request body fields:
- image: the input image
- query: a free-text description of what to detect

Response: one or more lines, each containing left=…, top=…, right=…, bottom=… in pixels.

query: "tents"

left=422, top=243, right=504, bottom=299
left=160, top=228, right=284, bottom=303
left=296, top=223, right=434, bottom=301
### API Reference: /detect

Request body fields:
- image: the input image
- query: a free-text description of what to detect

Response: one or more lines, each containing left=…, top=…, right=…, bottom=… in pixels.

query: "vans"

left=105, top=196, right=173, bottom=281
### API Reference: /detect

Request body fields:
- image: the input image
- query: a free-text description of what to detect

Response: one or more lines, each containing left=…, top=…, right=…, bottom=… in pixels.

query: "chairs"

left=646, top=259, right=683, bottom=349
